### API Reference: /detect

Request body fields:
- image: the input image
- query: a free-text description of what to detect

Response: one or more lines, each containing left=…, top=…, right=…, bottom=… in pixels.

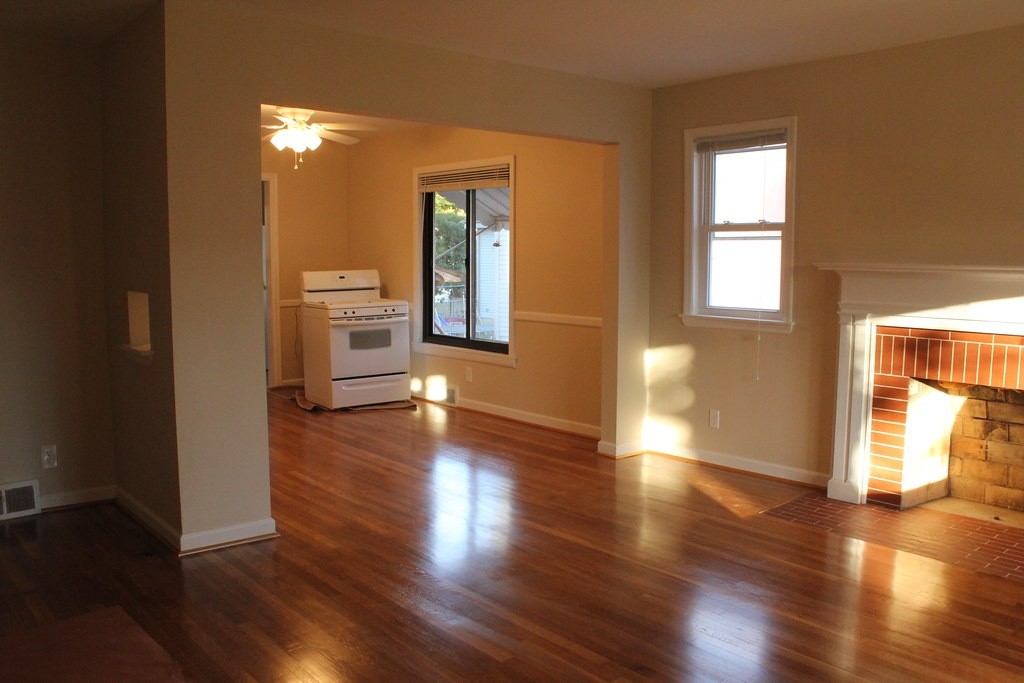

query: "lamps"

left=261, top=107, right=379, bottom=170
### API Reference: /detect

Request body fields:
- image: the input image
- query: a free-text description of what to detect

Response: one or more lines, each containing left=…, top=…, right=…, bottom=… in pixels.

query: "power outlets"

left=40, top=445, right=58, bottom=470
